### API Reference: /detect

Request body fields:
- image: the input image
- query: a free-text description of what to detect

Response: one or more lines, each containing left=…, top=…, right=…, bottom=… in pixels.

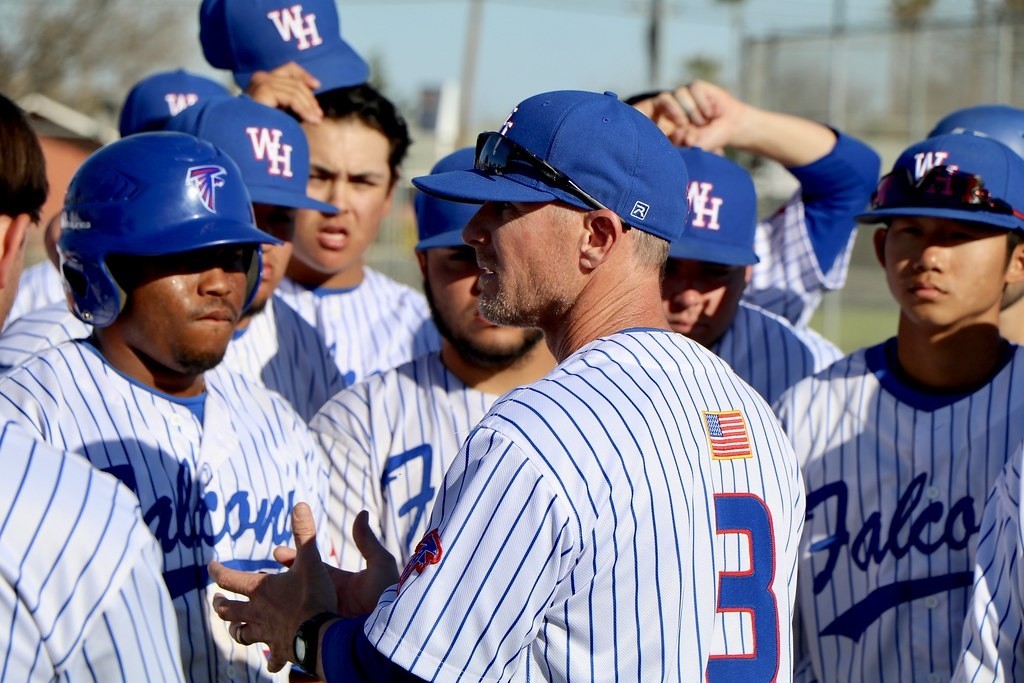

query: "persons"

left=949, top=438, right=1024, bottom=683
left=0, top=0, right=1023, bottom=683
left=770, top=132, right=1024, bottom=683
left=206, top=90, right=808, bottom=683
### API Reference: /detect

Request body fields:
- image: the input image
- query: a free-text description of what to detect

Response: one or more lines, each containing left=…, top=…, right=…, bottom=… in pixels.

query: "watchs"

left=293, top=611, right=346, bottom=679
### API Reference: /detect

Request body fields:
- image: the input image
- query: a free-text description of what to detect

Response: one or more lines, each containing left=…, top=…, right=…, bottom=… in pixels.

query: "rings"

left=235, top=624, right=249, bottom=645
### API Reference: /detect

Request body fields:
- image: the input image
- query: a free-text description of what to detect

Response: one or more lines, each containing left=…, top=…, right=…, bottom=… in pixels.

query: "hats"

left=118, top=69, right=231, bottom=139
left=169, top=93, right=340, bottom=213
left=199, top=0, right=371, bottom=96
left=414, top=147, right=484, bottom=250
left=926, top=105, right=1024, bottom=159
left=410, top=90, right=690, bottom=242
left=673, top=145, right=760, bottom=267
left=855, top=128, right=1024, bottom=228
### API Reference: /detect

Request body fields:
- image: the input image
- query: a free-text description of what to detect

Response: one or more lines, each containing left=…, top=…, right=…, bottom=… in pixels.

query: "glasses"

left=869, top=167, right=1024, bottom=220
left=474, top=131, right=631, bottom=229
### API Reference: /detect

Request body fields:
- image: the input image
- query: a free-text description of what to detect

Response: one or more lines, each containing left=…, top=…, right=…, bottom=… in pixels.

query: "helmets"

left=58, top=134, right=285, bottom=328
